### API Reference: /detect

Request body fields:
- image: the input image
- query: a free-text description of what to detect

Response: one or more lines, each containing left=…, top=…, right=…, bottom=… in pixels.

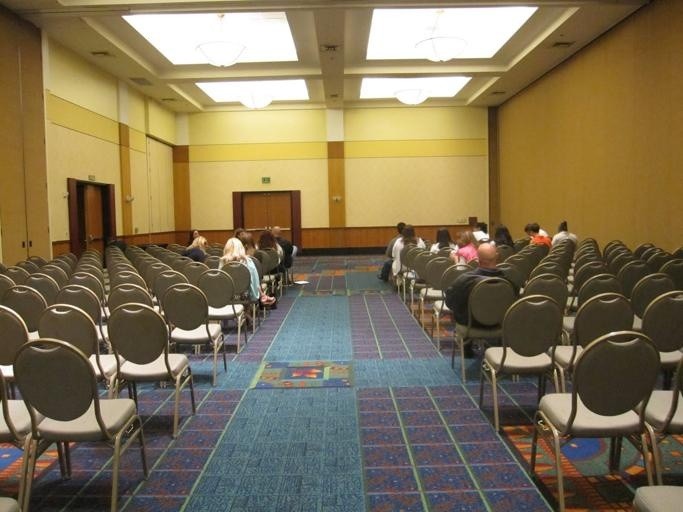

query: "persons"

left=390, top=225, right=426, bottom=278
left=430, top=227, right=459, bottom=260
left=447, top=220, right=577, bottom=272
left=377, top=222, right=408, bottom=280
left=444, top=243, right=520, bottom=360
left=189, top=230, right=200, bottom=243
left=215, top=225, right=294, bottom=329
left=182, top=235, right=208, bottom=263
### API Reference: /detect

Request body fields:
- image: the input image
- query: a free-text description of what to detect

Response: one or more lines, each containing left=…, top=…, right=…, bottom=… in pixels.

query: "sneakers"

left=261, top=296, right=276, bottom=304
